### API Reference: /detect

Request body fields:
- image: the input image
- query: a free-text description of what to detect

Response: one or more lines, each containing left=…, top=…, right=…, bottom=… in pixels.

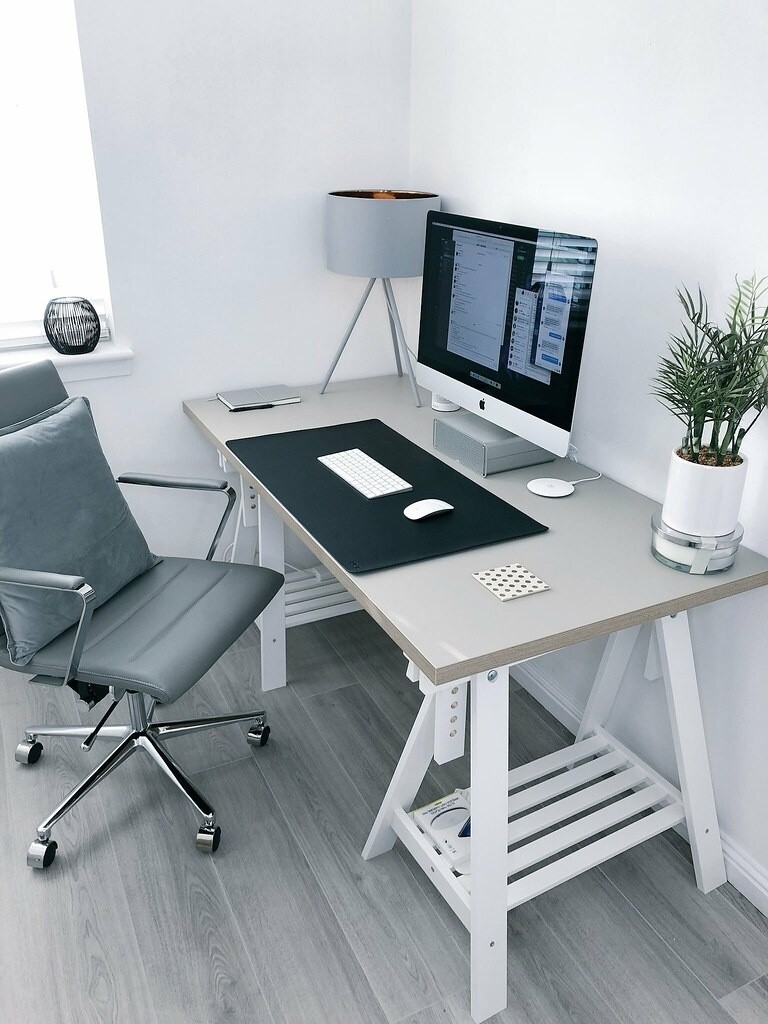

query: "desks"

left=182, top=372, right=768, bottom=1024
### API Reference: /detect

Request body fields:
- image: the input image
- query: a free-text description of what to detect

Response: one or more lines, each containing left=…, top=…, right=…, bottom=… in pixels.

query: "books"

left=217, top=384, right=301, bottom=410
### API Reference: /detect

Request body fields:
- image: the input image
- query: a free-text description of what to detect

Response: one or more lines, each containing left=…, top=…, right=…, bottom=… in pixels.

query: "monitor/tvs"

left=415, top=209, right=598, bottom=458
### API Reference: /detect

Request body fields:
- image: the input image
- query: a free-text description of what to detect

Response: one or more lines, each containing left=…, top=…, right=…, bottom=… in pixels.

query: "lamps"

left=318, top=189, right=441, bottom=409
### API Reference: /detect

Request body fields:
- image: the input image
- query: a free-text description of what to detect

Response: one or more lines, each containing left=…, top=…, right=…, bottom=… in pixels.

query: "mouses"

left=403, top=499, right=454, bottom=521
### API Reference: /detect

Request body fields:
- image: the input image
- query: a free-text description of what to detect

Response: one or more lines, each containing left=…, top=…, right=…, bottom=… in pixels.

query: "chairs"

left=0, top=359, right=286, bottom=868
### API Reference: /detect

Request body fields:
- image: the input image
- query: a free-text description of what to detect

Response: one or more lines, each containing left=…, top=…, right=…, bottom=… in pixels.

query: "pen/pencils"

left=229, top=404, right=273, bottom=412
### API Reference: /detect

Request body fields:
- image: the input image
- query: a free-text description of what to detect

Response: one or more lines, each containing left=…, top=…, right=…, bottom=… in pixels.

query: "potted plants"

left=649, top=269, right=768, bottom=573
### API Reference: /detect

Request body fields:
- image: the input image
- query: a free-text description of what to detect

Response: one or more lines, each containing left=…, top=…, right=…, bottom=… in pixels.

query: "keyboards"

left=316, top=448, right=413, bottom=500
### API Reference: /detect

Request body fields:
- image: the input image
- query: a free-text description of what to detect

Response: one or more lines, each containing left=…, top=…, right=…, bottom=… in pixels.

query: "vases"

left=43, top=296, right=102, bottom=357
